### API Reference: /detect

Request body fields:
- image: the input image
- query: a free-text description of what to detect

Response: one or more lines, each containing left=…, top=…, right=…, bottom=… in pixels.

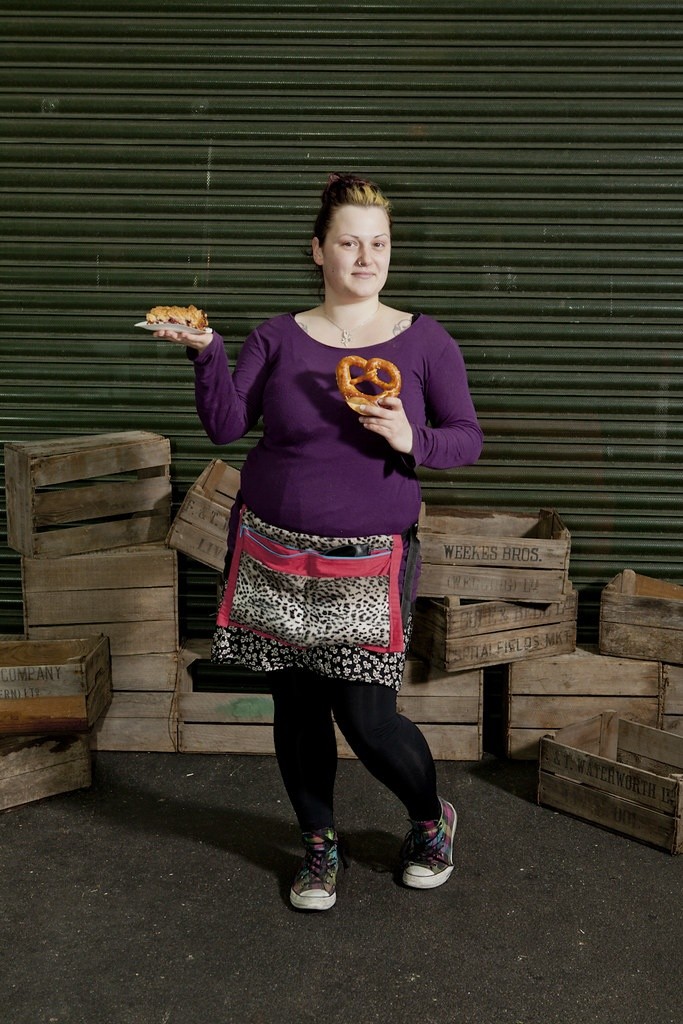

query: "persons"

left=151, top=172, right=484, bottom=911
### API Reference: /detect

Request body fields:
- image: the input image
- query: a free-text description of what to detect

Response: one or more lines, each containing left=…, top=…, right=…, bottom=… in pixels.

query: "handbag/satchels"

left=214, top=503, right=404, bottom=653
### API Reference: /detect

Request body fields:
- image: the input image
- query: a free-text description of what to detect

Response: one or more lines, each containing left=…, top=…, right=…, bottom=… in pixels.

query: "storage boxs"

left=597, top=567, right=683, bottom=666
left=407, top=588, right=579, bottom=675
left=175, top=638, right=278, bottom=758
left=20, top=539, right=182, bottom=659
left=506, top=643, right=662, bottom=762
left=332, top=659, right=487, bottom=764
left=0, top=734, right=93, bottom=813
left=165, top=457, right=241, bottom=575
left=663, top=662, right=683, bottom=737
left=534, top=707, right=683, bottom=858
left=0, top=633, right=114, bottom=734
left=3, top=430, right=172, bottom=560
left=79, top=649, right=178, bottom=756
left=413, top=498, right=572, bottom=604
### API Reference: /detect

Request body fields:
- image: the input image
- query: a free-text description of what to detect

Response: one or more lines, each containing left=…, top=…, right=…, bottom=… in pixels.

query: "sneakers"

left=290, top=828, right=348, bottom=909
left=400, top=797, right=457, bottom=889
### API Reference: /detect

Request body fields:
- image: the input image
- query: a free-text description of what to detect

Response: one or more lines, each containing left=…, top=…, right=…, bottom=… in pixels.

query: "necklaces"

left=319, top=300, right=380, bottom=347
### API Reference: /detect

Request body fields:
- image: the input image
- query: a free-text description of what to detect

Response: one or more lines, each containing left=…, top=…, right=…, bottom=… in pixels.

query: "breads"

left=336, top=355, right=402, bottom=416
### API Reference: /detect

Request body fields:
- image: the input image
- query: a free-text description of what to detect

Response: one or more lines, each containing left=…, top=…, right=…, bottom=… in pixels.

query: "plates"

left=134, top=322, right=212, bottom=335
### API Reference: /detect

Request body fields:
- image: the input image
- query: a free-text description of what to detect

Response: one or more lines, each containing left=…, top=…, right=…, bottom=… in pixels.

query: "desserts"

left=146, top=303, right=208, bottom=331
